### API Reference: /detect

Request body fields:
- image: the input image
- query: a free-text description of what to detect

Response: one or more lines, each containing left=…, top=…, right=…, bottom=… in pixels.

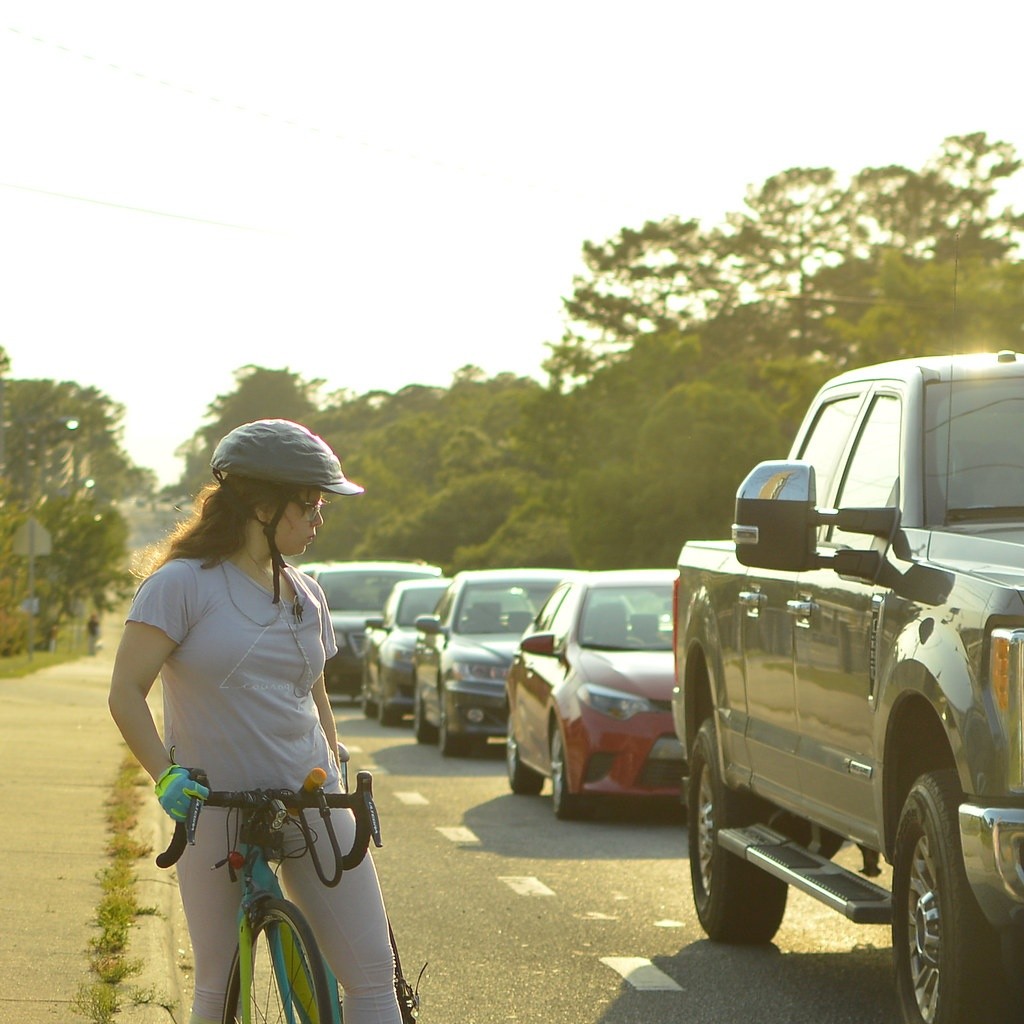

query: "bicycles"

left=153, top=758, right=428, bottom=1023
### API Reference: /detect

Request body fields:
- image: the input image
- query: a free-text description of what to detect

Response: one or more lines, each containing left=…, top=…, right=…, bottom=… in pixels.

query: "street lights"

left=25, top=415, right=82, bottom=662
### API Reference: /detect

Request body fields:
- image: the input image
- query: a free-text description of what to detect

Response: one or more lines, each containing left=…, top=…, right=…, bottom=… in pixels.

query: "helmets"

left=210, top=417, right=367, bottom=497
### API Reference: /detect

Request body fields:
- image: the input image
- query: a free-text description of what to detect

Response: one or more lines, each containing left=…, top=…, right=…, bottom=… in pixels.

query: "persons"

left=108, top=420, right=404, bottom=1024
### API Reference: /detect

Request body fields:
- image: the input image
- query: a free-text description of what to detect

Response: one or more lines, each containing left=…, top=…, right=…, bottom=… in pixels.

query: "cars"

left=408, top=569, right=590, bottom=753
left=356, top=579, right=455, bottom=725
left=502, top=566, right=688, bottom=820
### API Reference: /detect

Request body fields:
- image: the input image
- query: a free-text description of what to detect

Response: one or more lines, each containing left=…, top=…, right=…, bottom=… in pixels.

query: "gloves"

left=155, top=765, right=211, bottom=822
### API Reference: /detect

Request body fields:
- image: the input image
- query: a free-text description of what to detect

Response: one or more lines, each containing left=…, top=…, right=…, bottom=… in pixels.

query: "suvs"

left=298, top=559, right=449, bottom=689
left=669, top=349, right=1023, bottom=1024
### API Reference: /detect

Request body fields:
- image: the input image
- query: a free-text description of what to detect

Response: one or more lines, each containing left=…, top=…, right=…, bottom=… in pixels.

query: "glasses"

left=289, top=493, right=322, bottom=519
